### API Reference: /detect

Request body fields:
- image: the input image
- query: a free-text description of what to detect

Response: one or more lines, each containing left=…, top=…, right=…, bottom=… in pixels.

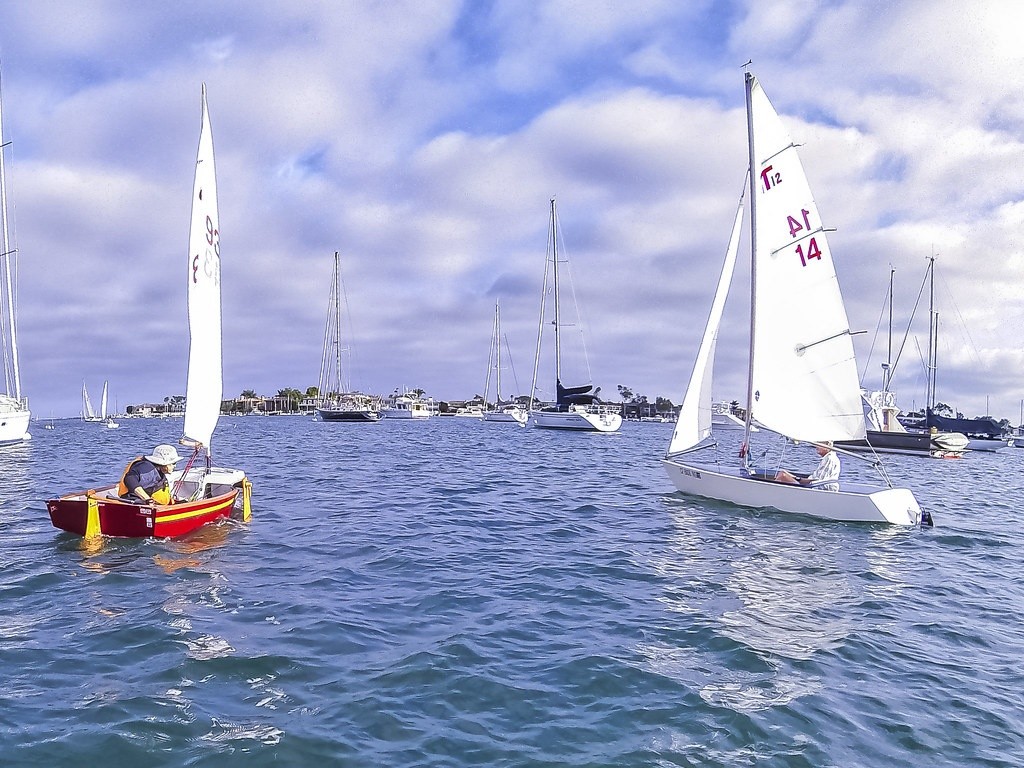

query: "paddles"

left=108, top=496, right=149, bottom=505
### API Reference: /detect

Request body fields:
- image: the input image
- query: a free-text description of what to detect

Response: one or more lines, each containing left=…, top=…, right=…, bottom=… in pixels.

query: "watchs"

left=795, top=476, right=801, bottom=482
left=146, top=498, right=153, bottom=505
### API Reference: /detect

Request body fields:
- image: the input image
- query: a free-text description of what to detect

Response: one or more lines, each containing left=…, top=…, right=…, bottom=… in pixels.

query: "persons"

left=118, top=445, right=184, bottom=505
left=775, top=441, right=840, bottom=492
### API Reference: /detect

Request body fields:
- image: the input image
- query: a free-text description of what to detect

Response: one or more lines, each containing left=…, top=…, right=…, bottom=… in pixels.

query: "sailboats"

left=480, top=297, right=530, bottom=424
left=811, top=243, right=973, bottom=460
left=527, top=196, right=622, bottom=433
left=660, top=58, right=934, bottom=529
left=80, top=379, right=120, bottom=429
left=314, top=251, right=387, bottom=421
left=1, top=92, right=31, bottom=444
left=44, top=82, right=248, bottom=539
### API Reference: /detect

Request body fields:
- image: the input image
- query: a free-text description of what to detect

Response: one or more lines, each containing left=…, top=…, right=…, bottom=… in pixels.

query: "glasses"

left=172, top=462, right=179, bottom=465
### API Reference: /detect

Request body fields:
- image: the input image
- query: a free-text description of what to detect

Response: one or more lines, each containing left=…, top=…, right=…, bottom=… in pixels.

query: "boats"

left=710, top=401, right=760, bottom=434
left=1013, top=435, right=1024, bottom=448
left=915, top=414, right=1009, bottom=452
left=454, top=405, right=485, bottom=418
left=381, top=393, right=431, bottom=419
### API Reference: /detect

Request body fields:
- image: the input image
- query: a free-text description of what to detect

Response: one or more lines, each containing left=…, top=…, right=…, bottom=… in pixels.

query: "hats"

left=144, top=445, right=184, bottom=466
